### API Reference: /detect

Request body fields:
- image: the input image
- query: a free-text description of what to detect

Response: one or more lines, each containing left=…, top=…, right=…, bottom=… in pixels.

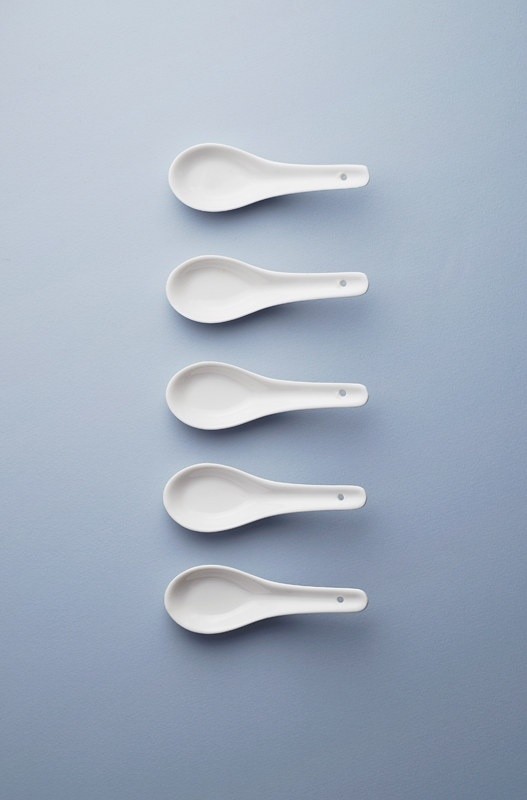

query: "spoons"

left=165, top=255, right=369, bottom=324
left=161, top=463, right=366, bottom=534
left=167, top=144, right=370, bottom=213
left=163, top=566, right=368, bottom=634
left=164, top=361, right=370, bottom=431
